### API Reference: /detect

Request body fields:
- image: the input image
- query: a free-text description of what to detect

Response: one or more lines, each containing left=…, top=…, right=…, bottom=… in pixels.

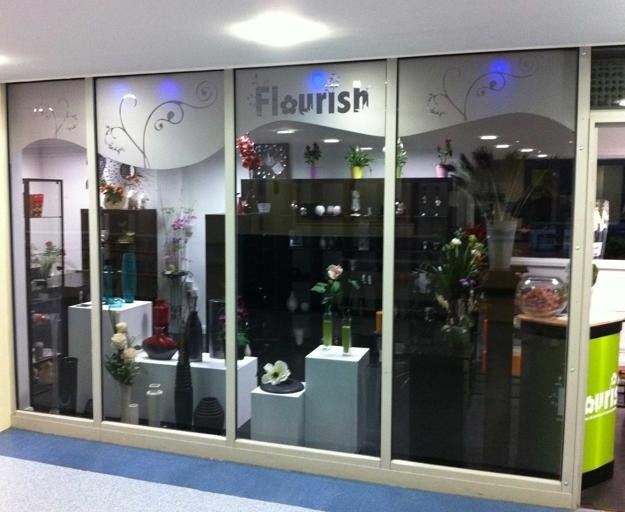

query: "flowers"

left=235, top=133, right=266, bottom=180
left=315, top=263, right=364, bottom=310
left=97, top=173, right=141, bottom=203
left=159, top=205, right=197, bottom=275
left=423, top=236, right=485, bottom=321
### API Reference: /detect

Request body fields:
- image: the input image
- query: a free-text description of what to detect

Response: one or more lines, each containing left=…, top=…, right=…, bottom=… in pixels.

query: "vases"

left=102, top=253, right=224, bottom=433
left=440, top=314, right=477, bottom=376
left=103, top=191, right=126, bottom=209
left=318, top=307, right=357, bottom=353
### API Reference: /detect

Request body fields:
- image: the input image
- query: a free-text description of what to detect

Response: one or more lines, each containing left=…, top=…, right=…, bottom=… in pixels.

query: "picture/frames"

left=250, top=143, right=290, bottom=179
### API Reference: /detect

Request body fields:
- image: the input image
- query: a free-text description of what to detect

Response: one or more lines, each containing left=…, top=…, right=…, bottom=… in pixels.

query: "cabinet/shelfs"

left=79, top=209, right=158, bottom=302
left=20, top=177, right=65, bottom=414
left=206, top=181, right=476, bottom=354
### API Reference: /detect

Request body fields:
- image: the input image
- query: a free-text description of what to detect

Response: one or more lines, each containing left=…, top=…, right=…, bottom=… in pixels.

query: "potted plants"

left=303, top=137, right=453, bottom=179
left=456, top=143, right=555, bottom=272
